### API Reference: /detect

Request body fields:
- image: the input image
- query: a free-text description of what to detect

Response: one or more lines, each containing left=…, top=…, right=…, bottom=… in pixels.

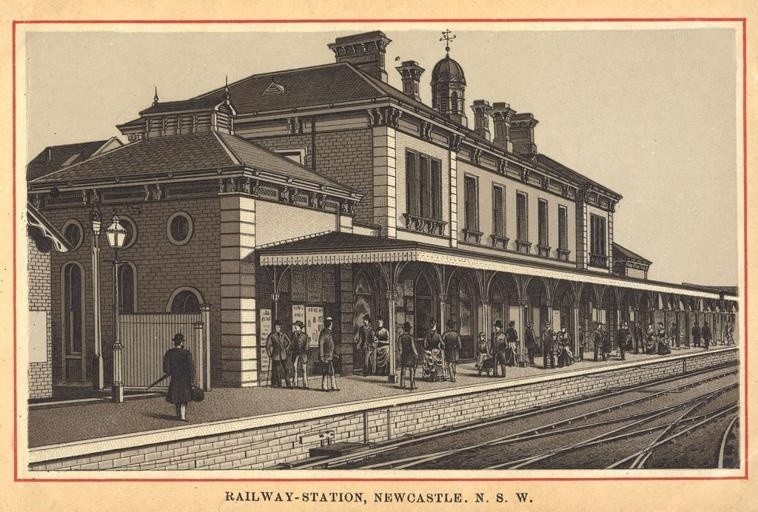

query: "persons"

left=160, top=333, right=197, bottom=423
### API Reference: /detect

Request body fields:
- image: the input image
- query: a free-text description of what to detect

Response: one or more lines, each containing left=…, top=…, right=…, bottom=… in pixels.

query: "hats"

left=172, top=333, right=185, bottom=342
left=292, top=321, right=306, bottom=327
left=446, top=319, right=459, bottom=325
left=362, top=314, right=372, bottom=321
left=402, top=322, right=412, bottom=329
left=493, top=320, right=504, bottom=328
left=544, top=322, right=552, bottom=327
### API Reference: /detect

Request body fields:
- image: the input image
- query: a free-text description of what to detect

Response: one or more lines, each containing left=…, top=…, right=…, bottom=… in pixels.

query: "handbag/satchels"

left=189, top=386, right=205, bottom=402
